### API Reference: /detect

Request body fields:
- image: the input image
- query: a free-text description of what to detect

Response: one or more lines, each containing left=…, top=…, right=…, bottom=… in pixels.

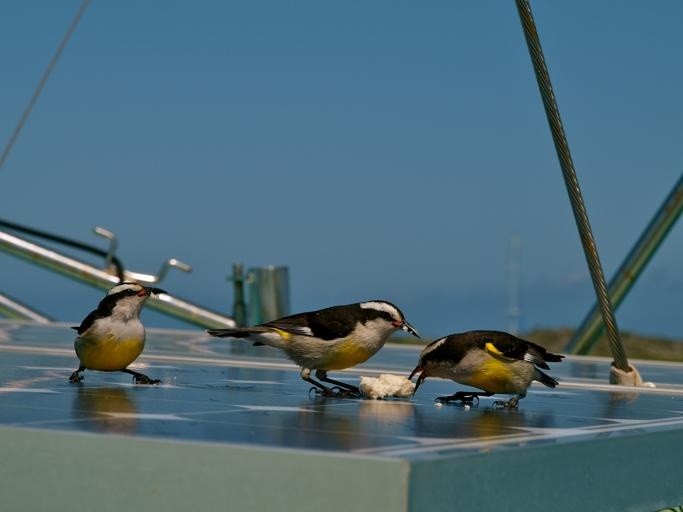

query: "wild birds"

left=408, top=329, right=567, bottom=411
left=68, top=282, right=169, bottom=385
left=204, top=299, right=421, bottom=399
